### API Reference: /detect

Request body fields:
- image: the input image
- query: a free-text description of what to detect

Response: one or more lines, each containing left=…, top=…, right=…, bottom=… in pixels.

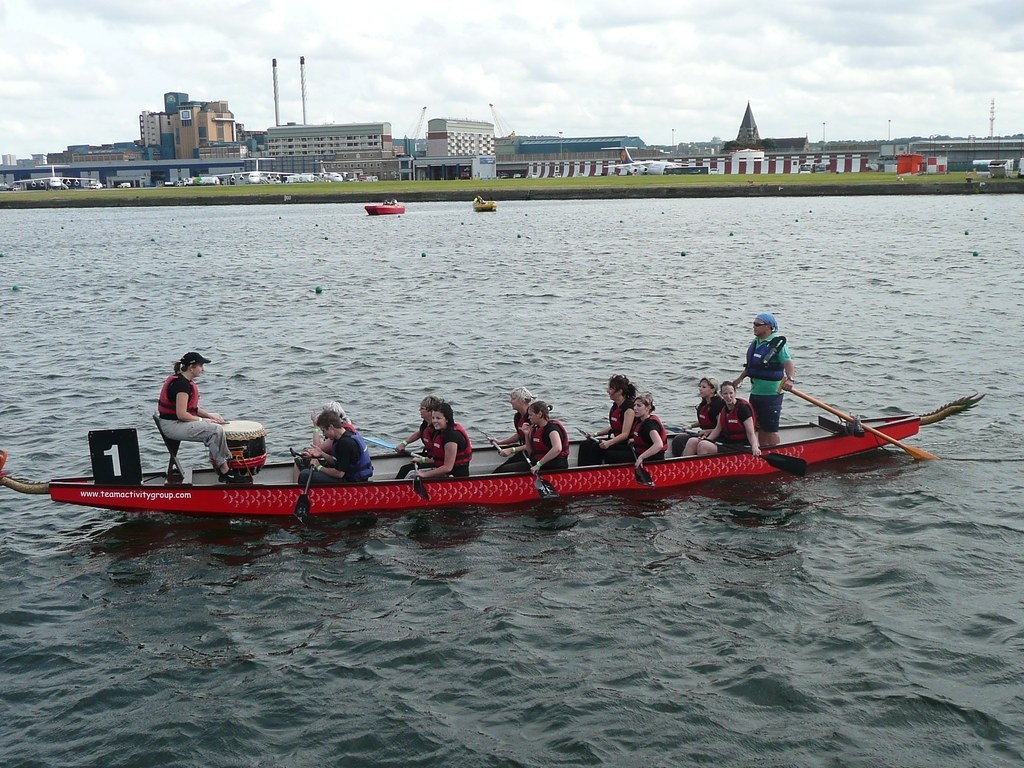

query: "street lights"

left=559, top=131, right=563, bottom=161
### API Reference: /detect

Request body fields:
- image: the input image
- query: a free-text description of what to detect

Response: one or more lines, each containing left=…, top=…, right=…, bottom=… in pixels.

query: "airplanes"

left=13, top=165, right=97, bottom=190
left=303, top=160, right=365, bottom=182
left=599, top=146, right=688, bottom=176
left=211, top=158, right=298, bottom=183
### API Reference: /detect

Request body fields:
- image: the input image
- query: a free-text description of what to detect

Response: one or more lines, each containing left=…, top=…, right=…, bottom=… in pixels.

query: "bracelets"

left=687, top=424, right=692, bottom=429
left=198, top=417, right=202, bottom=422
left=511, top=447, right=516, bottom=454
left=422, top=457, right=426, bottom=463
left=317, top=465, right=322, bottom=472
left=401, top=441, right=408, bottom=446
left=536, top=461, right=542, bottom=467
left=312, top=428, right=321, bottom=433
left=594, top=431, right=598, bottom=437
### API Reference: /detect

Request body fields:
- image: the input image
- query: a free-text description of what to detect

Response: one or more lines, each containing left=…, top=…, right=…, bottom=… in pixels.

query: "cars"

left=89, top=182, right=101, bottom=189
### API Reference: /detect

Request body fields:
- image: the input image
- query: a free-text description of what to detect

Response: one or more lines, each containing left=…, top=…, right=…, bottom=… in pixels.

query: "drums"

left=208, top=420, right=267, bottom=469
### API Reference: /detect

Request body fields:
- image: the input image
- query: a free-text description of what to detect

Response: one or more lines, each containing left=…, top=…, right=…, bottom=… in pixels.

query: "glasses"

left=753, top=322, right=767, bottom=327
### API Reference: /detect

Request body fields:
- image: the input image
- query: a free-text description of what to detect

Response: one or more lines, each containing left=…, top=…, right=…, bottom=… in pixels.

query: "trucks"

left=118, top=183, right=131, bottom=188
left=184, top=177, right=219, bottom=186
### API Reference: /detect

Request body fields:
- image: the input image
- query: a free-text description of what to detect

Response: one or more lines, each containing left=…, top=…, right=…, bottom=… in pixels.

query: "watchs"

left=787, top=375, right=794, bottom=382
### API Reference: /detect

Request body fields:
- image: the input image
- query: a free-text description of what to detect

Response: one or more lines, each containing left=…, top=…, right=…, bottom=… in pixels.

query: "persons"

left=672, top=377, right=725, bottom=457
left=490, top=387, right=569, bottom=475
left=473, top=195, right=482, bottom=206
left=469, top=170, right=472, bottom=180
left=341, top=172, right=344, bottom=181
left=157, top=352, right=249, bottom=483
left=683, top=381, right=761, bottom=456
left=578, top=374, right=638, bottom=466
left=383, top=198, right=398, bottom=206
left=605, top=395, right=668, bottom=469
left=395, top=396, right=472, bottom=480
left=293, top=402, right=374, bottom=484
left=733, top=313, right=794, bottom=445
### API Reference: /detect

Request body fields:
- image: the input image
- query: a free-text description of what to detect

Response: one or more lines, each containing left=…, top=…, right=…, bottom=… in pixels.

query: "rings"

left=396, top=449, right=398, bottom=451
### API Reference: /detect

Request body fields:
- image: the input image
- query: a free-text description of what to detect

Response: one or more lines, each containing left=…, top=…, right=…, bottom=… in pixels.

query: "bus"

left=710, top=168, right=719, bottom=174
left=360, top=175, right=378, bottom=181
left=797, top=163, right=832, bottom=174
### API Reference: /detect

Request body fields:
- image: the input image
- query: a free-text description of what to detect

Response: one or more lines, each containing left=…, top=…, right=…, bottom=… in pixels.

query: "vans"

left=1017, top=157, right=1024, bottom=177
left=175, top=181, right=184, bottom=187
left=13, top=185, right=22, bottom=190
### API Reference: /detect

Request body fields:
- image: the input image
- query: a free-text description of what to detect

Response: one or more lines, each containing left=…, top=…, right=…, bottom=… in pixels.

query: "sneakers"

left=218, top=467, right=252, bottom=483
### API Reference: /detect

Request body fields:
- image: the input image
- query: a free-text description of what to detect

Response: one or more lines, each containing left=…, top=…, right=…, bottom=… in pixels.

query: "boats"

left=365, top=205, right=406, bottom=216
left=473, top=201, right=497, bottom=212
left=0, top=391, right=988, bottom=511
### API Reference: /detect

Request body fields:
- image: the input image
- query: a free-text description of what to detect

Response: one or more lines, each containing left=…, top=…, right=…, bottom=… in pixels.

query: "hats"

left=756, top=312, right=778, bottom=333
left=180, top=352, right=211, bottom=366
left=701, top=376, right=719, bottom=393
left=633, top=392, right=655, bottom=411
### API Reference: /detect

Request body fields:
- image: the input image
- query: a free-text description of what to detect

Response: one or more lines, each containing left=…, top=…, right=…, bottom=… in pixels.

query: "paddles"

left=474, top=427, right=503, bottom=455
left=411, top=458, right=431, bottom=501
left=575, top=427, right=603, bottom=443
left=628, top=441, right=656, bottom=487
left=776, top=378, right=941, bottom=460
left=291, top=457, right=317, bottom=526
left=288, top=448, right=306, bottom=461
left=362, top=436, right=423, bottom=458
left=670, top=428, right=808, bottom=478
left=522, top=447, right=561, bottom=499
